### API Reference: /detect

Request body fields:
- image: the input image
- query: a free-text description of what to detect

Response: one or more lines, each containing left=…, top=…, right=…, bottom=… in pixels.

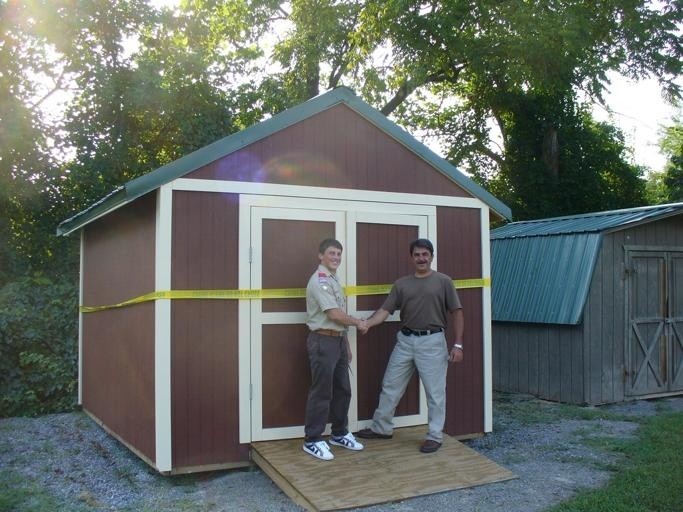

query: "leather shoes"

left=420, top=440, right=442, bottom=452
left=359, top=428, right=393, bottom=439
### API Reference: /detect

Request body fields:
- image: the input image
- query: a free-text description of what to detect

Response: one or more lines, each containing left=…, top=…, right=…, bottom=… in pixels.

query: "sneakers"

left=302, top=440, right=335, bottom=460
left=328, top=433, right=364, bottom=451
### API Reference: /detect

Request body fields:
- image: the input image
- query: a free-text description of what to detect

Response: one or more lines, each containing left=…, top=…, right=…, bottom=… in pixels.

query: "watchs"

left=453, top=343, right=464, bottom=350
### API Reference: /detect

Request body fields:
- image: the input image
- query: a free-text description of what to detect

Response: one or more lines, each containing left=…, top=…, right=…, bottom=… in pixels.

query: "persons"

left=358, top=239, right=464, bottom=453
left=303, top=239, right=369, bottom=461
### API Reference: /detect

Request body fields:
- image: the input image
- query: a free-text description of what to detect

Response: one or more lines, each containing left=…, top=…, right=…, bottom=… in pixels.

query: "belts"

left=316, top=328, right=347, bottom=338
left=402, top=327, right=443, bottom=336
